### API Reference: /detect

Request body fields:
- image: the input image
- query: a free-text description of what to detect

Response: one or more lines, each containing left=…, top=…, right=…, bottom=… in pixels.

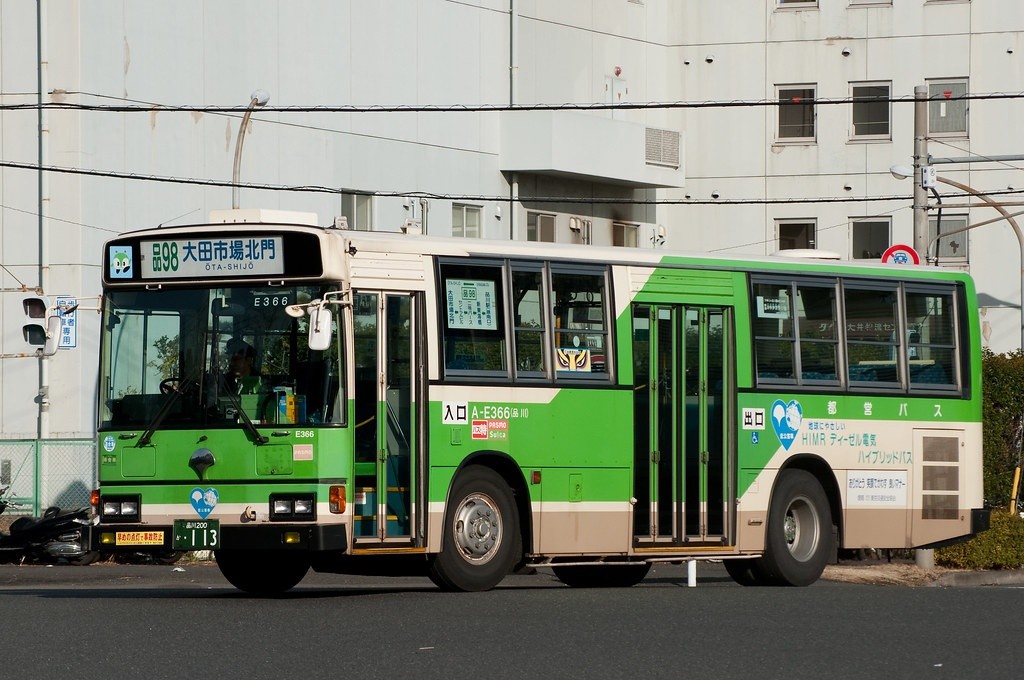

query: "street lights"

left=231, top=87, right=271, bottom=209
left=889, top=164, right=1023, bottom=355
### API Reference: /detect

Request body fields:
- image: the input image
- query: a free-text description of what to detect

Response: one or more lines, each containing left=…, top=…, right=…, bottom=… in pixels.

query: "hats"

left=225, top=338, right=256, bottom=357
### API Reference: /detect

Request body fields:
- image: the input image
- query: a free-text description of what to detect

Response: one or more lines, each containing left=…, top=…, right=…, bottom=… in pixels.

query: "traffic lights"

left=21, top=294, right=49, bottom=349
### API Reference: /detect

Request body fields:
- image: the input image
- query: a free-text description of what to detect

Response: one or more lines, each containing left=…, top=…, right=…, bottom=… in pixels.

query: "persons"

left=225, top=337, right=266, bottom=393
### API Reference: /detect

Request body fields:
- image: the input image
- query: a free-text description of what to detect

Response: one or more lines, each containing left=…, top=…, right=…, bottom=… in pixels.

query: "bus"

left=42, top=209, right=990, bottom=595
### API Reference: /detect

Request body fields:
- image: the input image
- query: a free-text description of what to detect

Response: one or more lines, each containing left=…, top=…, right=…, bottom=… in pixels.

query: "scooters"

left=0, top=484, right=186, bottom=567
